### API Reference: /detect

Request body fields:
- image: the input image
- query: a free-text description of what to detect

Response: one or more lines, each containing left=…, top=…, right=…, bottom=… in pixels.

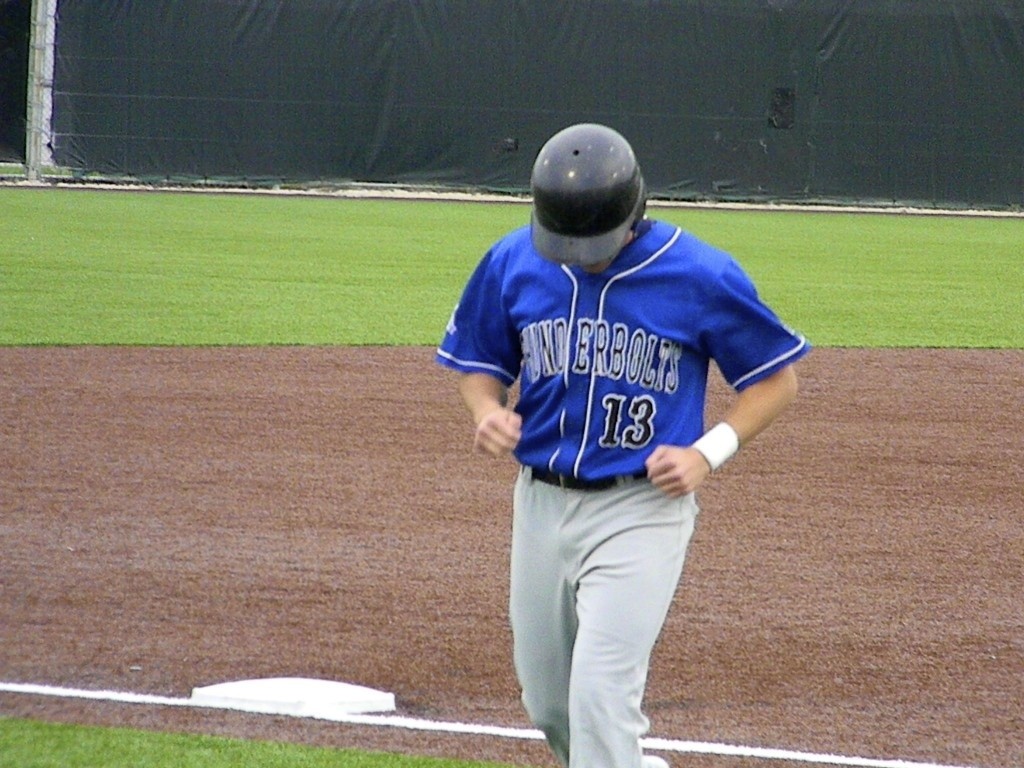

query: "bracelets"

left=693, top=421, right=739, bottom=475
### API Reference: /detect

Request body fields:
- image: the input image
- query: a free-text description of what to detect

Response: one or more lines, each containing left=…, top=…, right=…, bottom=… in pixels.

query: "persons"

left=437, top=123, right=812, bottom=768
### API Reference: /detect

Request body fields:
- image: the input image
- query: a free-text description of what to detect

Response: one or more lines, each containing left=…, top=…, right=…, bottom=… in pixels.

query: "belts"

left=531, top=465, right=649, bottom=491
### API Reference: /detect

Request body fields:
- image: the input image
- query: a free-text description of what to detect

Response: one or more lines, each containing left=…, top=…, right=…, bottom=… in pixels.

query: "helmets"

left=530, top=122, right=643, bottom=267
